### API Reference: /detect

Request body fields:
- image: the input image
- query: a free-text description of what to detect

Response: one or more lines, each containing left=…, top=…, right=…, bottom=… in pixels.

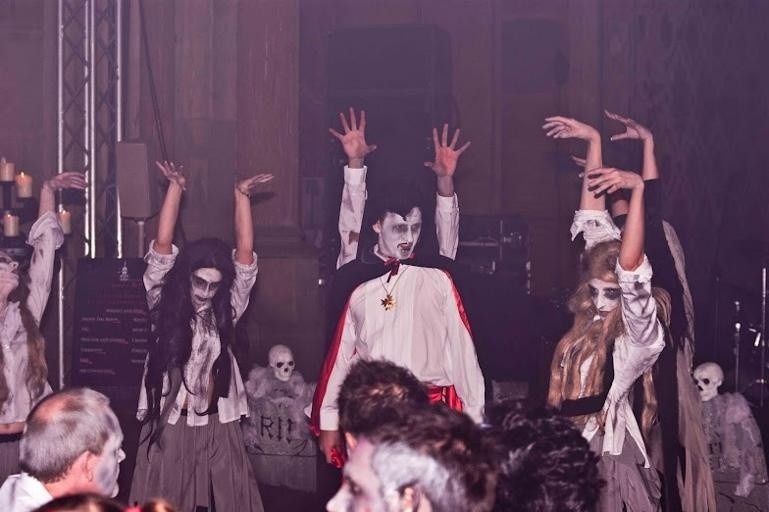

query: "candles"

left=0, top=156, right=33, bottom=199
left=3, top=214, right=19, bottom=238
left=57, top=210, right=72, bottom=236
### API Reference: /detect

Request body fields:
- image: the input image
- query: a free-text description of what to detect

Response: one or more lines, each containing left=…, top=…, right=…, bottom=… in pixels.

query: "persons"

left=693, top=362, right=769, bottom=512
